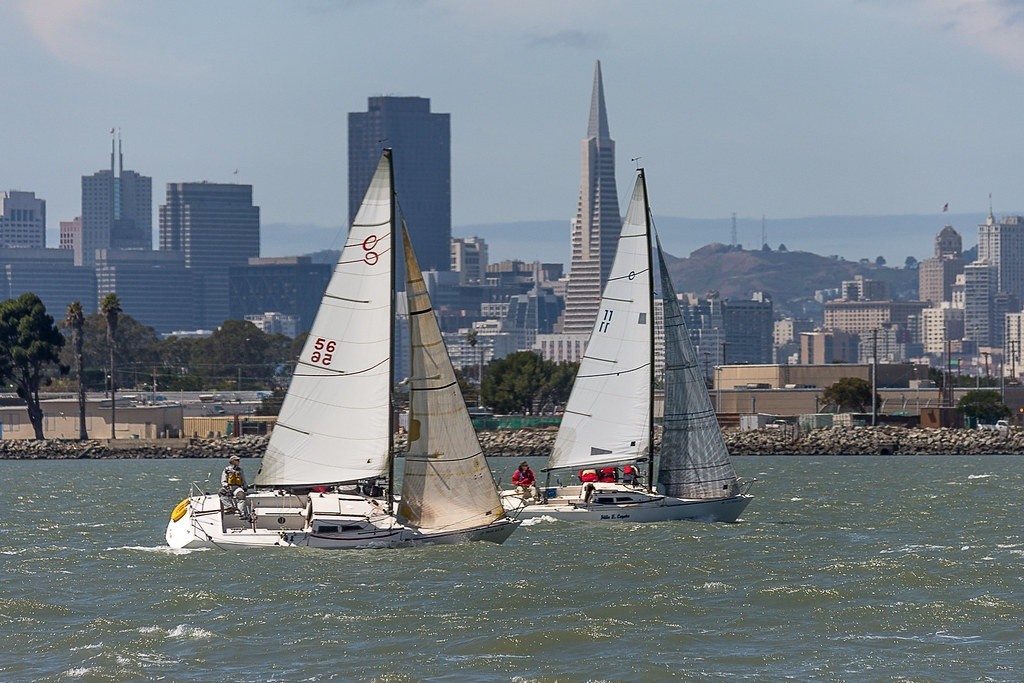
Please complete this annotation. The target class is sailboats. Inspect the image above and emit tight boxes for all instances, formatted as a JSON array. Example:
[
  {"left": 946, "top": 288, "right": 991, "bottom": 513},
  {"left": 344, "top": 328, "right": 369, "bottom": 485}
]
[
  {"left": 498, "top": 157, "right": 759, "bottom": 523},
  {"left": 166, "top": 139, "right": 530, "bottom": 552}
]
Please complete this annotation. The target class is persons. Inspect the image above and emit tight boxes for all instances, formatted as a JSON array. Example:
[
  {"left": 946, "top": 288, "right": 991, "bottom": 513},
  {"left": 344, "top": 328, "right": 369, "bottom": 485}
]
[
  {"left": 597, "top": 467, "right": 619, "bottom": 483},
  {"left": 218, "top": 455, "right": 246, "bottom": 519},
  {"left": 623, "top": 463, "right": 640, "bottom": 484},
  {"left": 578, "top": 468, "right": 600, "bottom": 483},
  {"left": 512, "top": 460, "right": 537, "bottom": 499}
]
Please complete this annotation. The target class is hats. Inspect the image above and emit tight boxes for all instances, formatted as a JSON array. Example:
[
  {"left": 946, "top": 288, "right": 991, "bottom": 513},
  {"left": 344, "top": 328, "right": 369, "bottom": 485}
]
[{"left": 229, "top": 455, "right": 240, "bottom": 463}]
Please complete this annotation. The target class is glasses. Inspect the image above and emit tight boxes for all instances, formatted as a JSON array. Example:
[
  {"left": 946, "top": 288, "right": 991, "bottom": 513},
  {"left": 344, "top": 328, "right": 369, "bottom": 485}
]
[
  {"left": 522, "top": 464, "right": 528, "bottom": 467},
  {"left": 234, "top": 459, "right": 240, "bottom": 461}
]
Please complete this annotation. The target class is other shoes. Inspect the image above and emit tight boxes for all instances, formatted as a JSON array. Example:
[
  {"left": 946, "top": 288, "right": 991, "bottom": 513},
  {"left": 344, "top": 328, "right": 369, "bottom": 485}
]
[{"left": 535, "top": 500, "right": 543, "bottom": 505}]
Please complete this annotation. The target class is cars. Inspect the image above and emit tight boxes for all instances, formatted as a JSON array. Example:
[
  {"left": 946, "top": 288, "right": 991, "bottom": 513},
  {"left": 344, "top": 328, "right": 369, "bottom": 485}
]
[{"left": 147, "top": 396, "right": 167, "bottom": 406}]
